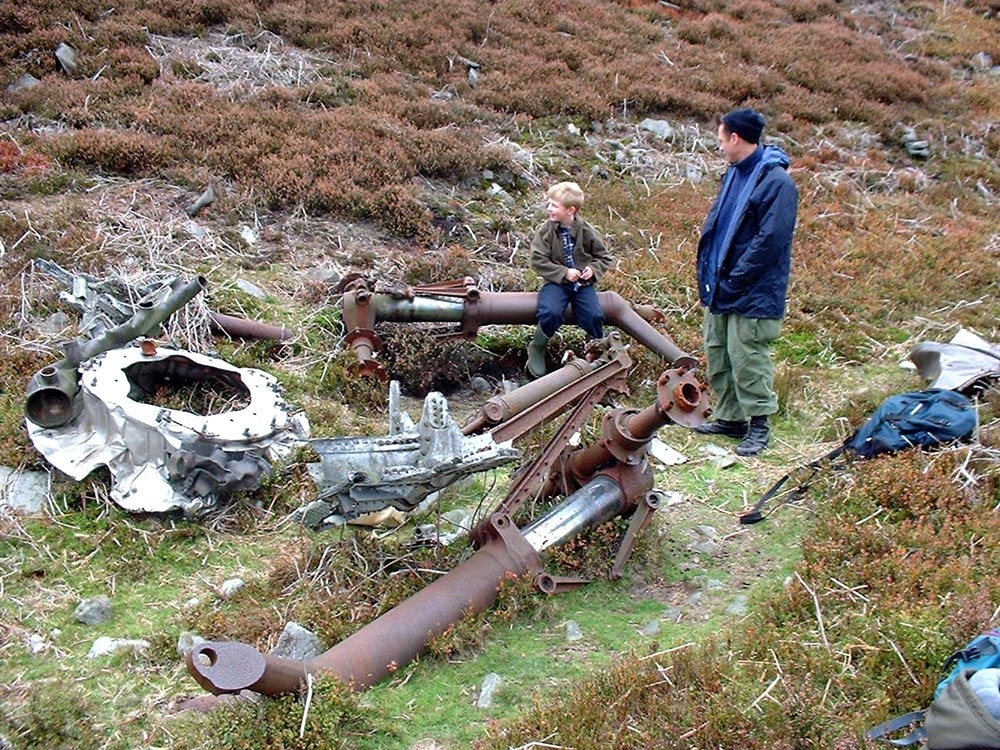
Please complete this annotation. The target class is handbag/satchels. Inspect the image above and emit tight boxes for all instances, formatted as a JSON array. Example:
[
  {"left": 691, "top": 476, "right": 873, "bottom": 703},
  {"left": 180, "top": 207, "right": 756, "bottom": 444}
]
[{"left": 847, "top": 388, "right": 976, "bottom": 459}]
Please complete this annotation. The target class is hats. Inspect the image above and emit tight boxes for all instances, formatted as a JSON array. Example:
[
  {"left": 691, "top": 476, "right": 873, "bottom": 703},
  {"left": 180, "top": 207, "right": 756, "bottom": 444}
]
[{"left": 723, "top": 110, "right": 767, "bottom": 144}]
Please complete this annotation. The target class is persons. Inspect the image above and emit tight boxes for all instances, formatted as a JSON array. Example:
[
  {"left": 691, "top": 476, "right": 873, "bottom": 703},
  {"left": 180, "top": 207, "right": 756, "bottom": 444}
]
[
  {"left": 692, "top": 108, "right": 799, "bottom": 457},
  {"left": 527, "top": 182, "right": 612, "bottom": 378}
]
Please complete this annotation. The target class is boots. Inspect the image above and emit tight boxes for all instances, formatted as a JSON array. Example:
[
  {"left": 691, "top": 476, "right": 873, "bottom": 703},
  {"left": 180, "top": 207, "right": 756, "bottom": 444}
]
[{"left": 527, "top": 321, "right": 552, "bottom": 378}]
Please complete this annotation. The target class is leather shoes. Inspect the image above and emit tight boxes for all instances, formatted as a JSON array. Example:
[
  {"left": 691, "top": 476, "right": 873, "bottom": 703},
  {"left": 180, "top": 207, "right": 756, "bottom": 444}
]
[
  {"left": 734, "top": 421, "right": 769, "bottom": 455},
  {"left": 692, "top": 417, "right": 748, "bottom": 438}
]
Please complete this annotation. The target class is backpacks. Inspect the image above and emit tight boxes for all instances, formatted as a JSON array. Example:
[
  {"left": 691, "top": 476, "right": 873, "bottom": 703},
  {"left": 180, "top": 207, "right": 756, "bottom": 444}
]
[{"left": 922, "top": 625, "right": 1000, "bottom": 750}]
